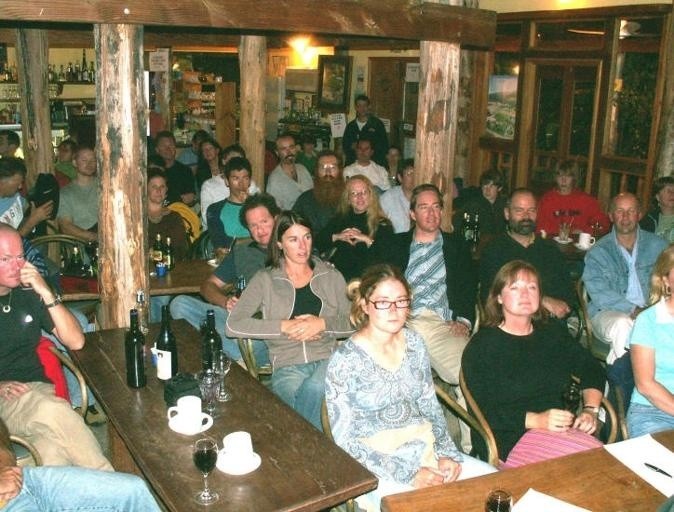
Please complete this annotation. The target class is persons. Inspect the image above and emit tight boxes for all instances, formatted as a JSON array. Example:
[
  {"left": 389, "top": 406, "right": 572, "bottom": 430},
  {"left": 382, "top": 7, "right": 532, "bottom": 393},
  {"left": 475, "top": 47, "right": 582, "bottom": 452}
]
[
  {"left": 0, "top": 129, "right": 20, "bottom": 161},
  {"left": 0, "top": 416, "right": 163, "bottom": 510},
  {"left": 0, "top": 156, "right": 54, "bottom": 281},
  {"left": 341, "top": 94, "right": 389, "bottom": 169},
  {"left": 0, "top": 222, "right": 115, "bottom": 474}
]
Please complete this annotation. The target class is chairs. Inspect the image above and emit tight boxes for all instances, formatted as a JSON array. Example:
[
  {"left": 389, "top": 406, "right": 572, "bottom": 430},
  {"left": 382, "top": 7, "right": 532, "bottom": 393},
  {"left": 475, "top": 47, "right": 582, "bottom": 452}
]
[{"left": 0, "top": 220, "right": 674, "bottom": 512}]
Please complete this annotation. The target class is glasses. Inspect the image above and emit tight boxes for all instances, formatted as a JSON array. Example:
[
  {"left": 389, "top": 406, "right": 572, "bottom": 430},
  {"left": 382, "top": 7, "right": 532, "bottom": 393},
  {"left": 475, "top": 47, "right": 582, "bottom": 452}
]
[{"left": 365, "top": 296, "right": 414, "bottom": 311}]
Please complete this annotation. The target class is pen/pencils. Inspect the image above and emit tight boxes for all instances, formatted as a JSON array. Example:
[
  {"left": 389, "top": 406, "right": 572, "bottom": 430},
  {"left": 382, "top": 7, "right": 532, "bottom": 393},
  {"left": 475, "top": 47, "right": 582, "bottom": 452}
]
[{"left": 644, "top": 463, "right": 673, "bottom": 479}]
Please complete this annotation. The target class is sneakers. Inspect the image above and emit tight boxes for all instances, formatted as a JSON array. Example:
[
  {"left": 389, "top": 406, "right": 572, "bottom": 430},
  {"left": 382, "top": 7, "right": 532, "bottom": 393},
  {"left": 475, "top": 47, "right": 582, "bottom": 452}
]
[{"left": 74, "top": 406, "right": 107, "bottom": 427}]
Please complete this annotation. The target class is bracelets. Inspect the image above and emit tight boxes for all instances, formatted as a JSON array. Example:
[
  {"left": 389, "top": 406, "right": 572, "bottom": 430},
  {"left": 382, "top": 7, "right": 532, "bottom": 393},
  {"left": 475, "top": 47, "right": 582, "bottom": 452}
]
[{"left": 42, "top": 291, "right": 62, "bottom": 310}]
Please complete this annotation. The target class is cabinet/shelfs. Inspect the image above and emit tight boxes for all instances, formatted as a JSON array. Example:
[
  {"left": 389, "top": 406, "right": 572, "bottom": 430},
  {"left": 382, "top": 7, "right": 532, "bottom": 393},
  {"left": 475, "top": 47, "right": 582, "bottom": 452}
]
[{"left": 178, "top": 77, "right": 237, "bottom": 150}]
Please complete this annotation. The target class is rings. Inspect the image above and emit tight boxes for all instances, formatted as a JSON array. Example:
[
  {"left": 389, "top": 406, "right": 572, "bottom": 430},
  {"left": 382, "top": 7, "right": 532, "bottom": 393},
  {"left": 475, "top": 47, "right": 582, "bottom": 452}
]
[{"left": 5, "top": 387, "right": 10, "bottom": 392}]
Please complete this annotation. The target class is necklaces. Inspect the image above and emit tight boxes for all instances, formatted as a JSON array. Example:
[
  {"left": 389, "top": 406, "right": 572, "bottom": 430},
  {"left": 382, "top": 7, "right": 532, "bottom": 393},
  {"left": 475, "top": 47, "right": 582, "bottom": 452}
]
[{"left": 0, "top": 288, "right": 11, "bottom": 313}]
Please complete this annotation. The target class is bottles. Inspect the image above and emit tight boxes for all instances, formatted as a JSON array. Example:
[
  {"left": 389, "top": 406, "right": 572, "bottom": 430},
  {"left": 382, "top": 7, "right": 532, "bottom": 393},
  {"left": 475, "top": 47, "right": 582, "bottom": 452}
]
[
  {"left": 236, "top": 275, "right": 246, "bottom": 298},
  {"left": 57, "top": 231, "right": 175, "bottom": 278},
  {"left": 155, "top": 305, "right": 178, "bottom": 379},
  {"left": 125, "top": 311, "right": 145, "bottom": 388},
  {"left": 134, "top": 292, "right": 149, "bottom": 334},
  {"left": 559, "top": 382, "right": 581, "bottom": 427},
  {"left": 460, "top": 211, "right": 480, "bottom": 244}
]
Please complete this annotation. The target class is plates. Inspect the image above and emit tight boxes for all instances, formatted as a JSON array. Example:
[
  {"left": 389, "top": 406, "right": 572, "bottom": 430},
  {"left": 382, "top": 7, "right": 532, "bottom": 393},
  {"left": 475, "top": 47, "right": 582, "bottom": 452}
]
[
  {"left": 168, "top": 412, "right": 211, "bottom": 435},
  {"left": 575, "top": 242, "right": 589, "bottom": 251},
  {"left": 206, "top": 258, "right": 219, "bottom": 269},
  {"left": 216, "top": 451, "right": 263, "bottom": 474},
  {"left": 554, "top": 236, "right": 574, "bottom": 244}
]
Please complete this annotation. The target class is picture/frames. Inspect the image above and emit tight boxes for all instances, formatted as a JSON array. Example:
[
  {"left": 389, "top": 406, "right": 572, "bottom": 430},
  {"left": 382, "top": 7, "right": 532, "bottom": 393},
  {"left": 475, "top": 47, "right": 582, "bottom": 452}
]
[{"left": 316, "top": 53, "right": 354, "bottom": 116}]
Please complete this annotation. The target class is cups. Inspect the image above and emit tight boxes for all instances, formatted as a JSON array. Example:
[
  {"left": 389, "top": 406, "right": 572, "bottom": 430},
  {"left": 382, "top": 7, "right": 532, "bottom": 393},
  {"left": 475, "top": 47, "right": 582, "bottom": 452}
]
[
  {"left": 164, "top": 396, "right": 204, "bottom": 428},
  {"left": 539, "top": 231, "right": 547, "bottom": 240},
  {"left": 579, "top": 232, "right": 597, "bottom": 248},
  {"left": 484, "top": 487, "right": 513, "bottom": 511},
  {"left": 560, "top": 222, "right": 570, "bottom": 240},
  {"left": 223, "top": 431, "right": 254, "bottom": 465}
]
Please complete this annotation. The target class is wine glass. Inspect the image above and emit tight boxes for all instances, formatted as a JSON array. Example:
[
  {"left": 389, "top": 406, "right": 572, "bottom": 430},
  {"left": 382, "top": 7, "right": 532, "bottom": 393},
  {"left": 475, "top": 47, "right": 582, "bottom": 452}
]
[
  {"left": 192, "top": 440, "right": 219, "bottom": 507},
  {"left": 199, "top": 310, "right": 231, "bottom": 413}
]
